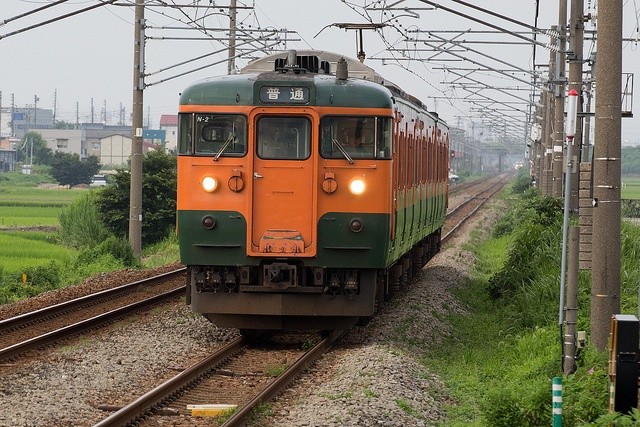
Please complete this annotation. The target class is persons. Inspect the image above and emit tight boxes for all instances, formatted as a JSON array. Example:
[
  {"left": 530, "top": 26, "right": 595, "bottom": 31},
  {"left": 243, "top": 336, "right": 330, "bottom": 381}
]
[{"left": 346, "top": 127, "right": 363, "bottom": 148}]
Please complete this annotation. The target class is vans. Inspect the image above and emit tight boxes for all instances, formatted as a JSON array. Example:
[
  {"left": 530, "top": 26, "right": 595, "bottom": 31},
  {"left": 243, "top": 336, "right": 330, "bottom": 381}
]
[{"left": 89, "top": 175, "right": 108, "bottom": 188}]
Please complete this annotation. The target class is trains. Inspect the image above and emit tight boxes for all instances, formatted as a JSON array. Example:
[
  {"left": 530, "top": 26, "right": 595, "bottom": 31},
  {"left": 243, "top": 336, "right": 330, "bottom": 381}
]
[{"left": 178, "top": 49, "right": 449, "bottom": 334}]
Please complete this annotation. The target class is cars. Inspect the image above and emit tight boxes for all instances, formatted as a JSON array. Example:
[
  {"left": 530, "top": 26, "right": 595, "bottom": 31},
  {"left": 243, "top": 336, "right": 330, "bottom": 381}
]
[{"left": 449, "top": 173, "right": 459, "bottom": 182}]
